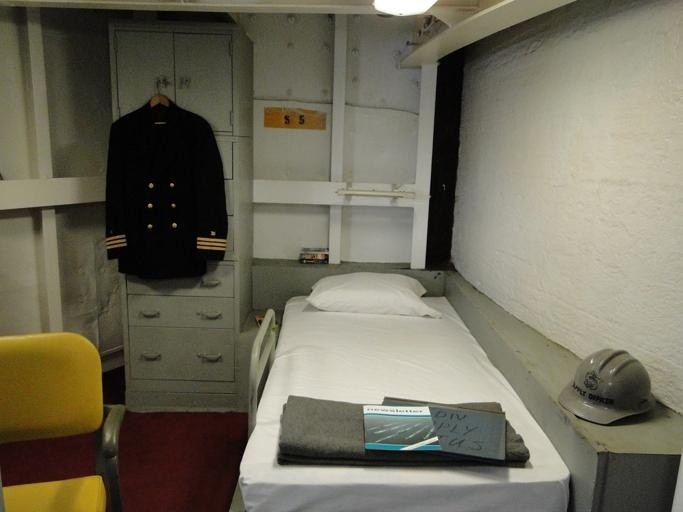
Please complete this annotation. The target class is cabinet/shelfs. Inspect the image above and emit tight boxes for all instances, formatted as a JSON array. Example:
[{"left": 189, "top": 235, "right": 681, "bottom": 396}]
[
  {"left": 108, "top": 21, "right": 253, "bottom": 413},
  {"left": 0, "top": 332, "right": 125, "bottom": 512}
]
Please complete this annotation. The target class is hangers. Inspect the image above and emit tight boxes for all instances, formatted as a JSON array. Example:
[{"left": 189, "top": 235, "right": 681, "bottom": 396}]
[{"left": 148, "top": 77, "right": 169, "bottom": 110}]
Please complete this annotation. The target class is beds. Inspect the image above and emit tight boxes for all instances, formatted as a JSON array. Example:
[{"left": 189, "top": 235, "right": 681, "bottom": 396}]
[{"left": 237, "top": 295, "right": 571, "bottom": 511}]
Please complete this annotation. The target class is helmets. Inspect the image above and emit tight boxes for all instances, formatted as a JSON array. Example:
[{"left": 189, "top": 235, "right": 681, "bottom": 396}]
[{"left": 557, "top": 348, "right": 658, "bottom": 427}]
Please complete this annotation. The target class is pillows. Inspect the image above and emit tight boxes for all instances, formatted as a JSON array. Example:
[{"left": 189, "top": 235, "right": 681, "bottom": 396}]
[{"left": 305, "top": 270, "right": 439, "bottom": 318}]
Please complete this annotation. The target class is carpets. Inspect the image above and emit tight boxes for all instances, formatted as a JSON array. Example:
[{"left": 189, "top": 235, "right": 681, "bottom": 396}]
[{"left": 0, "top": 412, "right": 248, "bottom": 512}]
